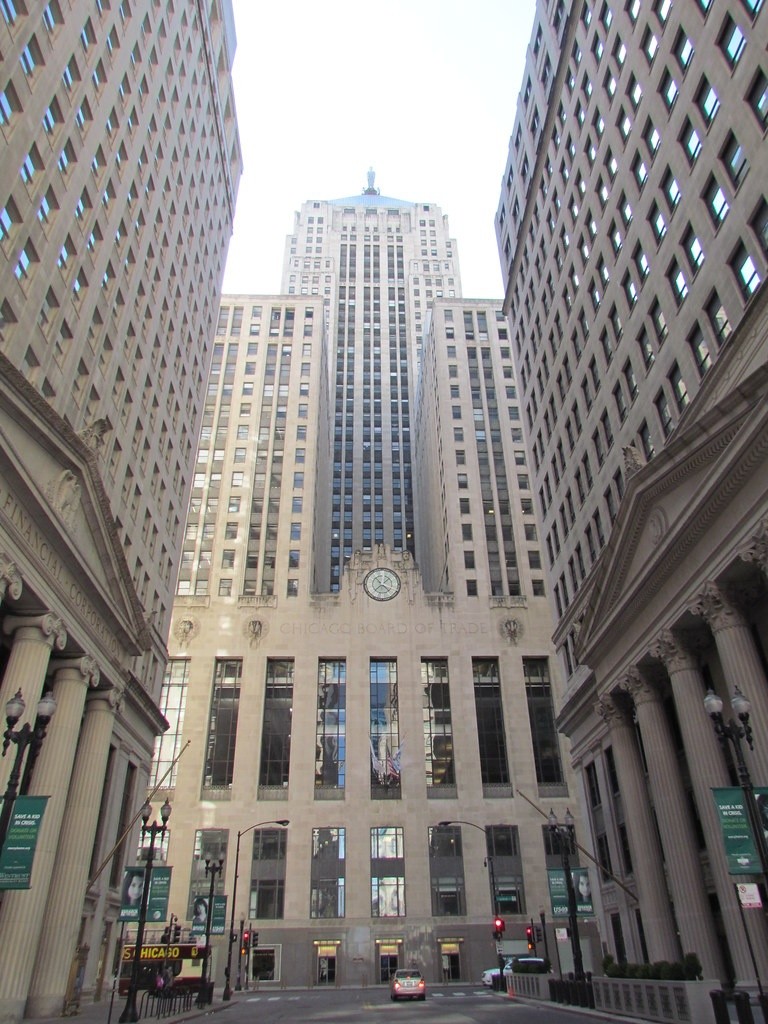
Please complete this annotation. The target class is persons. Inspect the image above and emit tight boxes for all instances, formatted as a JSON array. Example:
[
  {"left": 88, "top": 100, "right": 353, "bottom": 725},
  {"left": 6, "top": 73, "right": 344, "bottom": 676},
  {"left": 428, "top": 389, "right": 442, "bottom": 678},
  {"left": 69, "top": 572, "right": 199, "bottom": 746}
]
[
  {"left": 193, "top": 898, "right": 207, "bottom": 926},
  {"left": 573, "top": 872, "right": 592, "bottom": 904},
  {"left": 122, "top": 870, "right": 144, "bottom": 905},
  {"left": 411, "top": 972, "right": 417, "bottom": 976},
  {"left": 156, "top": 964, "right": 173, "bottom": 997}
]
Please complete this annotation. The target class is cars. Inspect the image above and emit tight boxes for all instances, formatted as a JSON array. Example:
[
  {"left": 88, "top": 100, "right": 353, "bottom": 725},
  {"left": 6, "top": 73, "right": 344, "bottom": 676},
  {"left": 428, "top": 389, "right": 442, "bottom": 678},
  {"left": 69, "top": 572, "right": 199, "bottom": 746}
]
[{"left": 388, "top": 968, "right": 426, "bottom": 1001}]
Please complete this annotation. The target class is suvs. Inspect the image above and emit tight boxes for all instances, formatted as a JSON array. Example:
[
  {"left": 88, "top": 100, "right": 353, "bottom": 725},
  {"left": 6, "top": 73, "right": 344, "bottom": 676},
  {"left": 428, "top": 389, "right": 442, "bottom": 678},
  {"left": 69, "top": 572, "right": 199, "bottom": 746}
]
[{"left": 480, "top": 957, "right": 555, "bottom": 989}]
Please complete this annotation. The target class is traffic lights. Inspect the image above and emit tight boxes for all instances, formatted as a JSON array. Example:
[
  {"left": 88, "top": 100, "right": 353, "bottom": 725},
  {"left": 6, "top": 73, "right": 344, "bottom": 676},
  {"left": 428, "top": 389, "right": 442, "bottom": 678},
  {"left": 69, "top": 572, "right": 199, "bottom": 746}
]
[
  {"left": 243, "top": 931, "right": 249, "bottom": 947},
  {"left": 528, "top": 942, "right": 535, "bottom": 950},
  {"left": 253, "top": 932, "right": 259, "bottom": 946},
  {"left": 495, "top": 918, "right": 502, "bottom": 933},
  {"left": 526, "top": 927, "right": 533, "bottom": 942},
  {"left": 533, "top": 925, "right": 543, "bottom": 943}
]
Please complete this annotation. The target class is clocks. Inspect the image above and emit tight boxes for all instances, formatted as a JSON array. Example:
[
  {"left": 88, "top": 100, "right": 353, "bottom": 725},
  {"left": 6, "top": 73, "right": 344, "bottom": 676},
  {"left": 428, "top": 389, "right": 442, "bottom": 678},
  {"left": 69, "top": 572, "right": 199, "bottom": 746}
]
[{"left": 362, "top": 567, "right": 402, "bottom": 601}]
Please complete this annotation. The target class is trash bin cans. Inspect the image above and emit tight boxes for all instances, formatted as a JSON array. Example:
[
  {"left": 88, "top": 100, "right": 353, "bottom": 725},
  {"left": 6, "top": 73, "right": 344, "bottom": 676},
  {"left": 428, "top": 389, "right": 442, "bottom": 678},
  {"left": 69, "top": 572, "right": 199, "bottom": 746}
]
[
  {"left": 492, "top": 974, "right": 505, "bottom": 991},
  {"left": 195, "top": 982, "right": 214, "bottom": 1005}
]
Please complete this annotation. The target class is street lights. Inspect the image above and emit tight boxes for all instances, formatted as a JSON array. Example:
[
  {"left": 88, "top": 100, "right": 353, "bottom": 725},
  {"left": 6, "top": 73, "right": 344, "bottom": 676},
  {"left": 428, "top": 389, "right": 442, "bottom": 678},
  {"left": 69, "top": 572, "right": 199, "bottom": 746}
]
[
  {"left": 702, "top": 684, "right": 768, "bottom": 897},
  {"left": 117, "top": 797, "right": 173, "bottom": 1024},
  {"left": 222, "top": 819, "right": 290, "bottom": 1001},
  {"left": 438, "top": 820, "right": 505, "bottom": 990},
  {"left": 173, "top": 924, "right": 181, "bottom": 943},
  {"left": 194, "top": 850, "right": 226, "bottom": 1004},
  {"left": 546, "top": 806, "right": 588, "bottom": 1008},
  {"left": 0, "top": 686, "right": 59, "bottom": 860}
]
